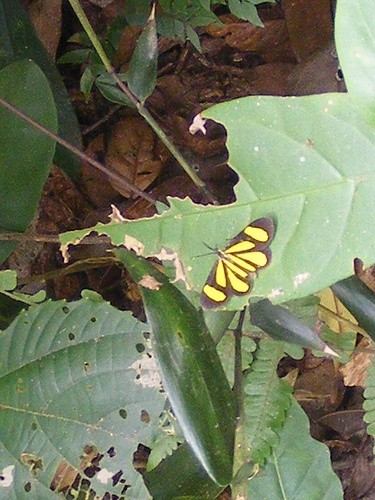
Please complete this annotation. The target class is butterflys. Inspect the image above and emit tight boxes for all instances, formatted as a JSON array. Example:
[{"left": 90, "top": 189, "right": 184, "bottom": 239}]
[{"left": 191, "top": 217, "right": 275, "bottom": 309}]
